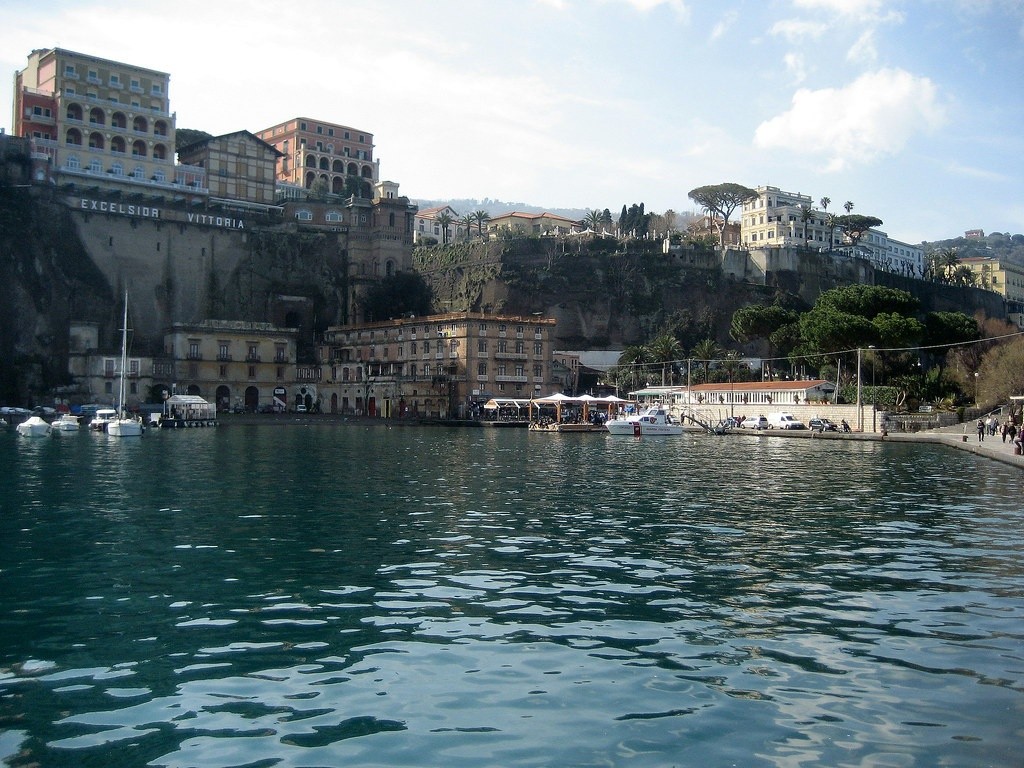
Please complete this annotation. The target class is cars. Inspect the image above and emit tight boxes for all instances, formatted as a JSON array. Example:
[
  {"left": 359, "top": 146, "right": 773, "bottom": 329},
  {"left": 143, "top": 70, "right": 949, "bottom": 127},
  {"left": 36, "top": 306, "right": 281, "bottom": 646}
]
[
  {"left": 725, "top": 417, "right": 740, "bottom": 427},
  {"left": 262, "top": 405, "right": 274, "bottom": 414},
  {"left": 297, "top": 405, "right": 307, "bottom": 413},
  {"left": 809, "top": 419, "right": 838, "bottom": 432}
]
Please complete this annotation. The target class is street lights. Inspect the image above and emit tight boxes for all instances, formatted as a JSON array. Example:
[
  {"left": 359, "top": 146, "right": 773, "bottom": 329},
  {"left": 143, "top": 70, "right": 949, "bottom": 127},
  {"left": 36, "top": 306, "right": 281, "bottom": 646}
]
[
  {"left": 973, "top": 372, "right": 979, "bottom": 396},
  {"left": 868, "top": 345, "right": 877, "bottom": 432}
]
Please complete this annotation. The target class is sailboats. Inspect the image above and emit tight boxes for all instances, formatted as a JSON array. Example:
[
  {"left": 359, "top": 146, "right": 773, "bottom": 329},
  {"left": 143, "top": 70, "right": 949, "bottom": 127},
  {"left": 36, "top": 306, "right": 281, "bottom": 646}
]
[{"left": 108, "top": 293, "right": 146, "bottom": 436}]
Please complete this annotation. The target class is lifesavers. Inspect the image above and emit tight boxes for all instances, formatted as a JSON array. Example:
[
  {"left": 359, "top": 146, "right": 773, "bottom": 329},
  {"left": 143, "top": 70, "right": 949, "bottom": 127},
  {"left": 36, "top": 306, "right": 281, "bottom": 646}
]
[{"left": 649, "top": 417, "right": 655, "bottom": 423}]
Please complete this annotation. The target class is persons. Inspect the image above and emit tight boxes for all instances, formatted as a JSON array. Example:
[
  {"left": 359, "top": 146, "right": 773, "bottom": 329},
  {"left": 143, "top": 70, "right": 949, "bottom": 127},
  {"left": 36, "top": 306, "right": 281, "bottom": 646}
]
[
  {"left": 739, "top": 416, "right": 745, "bottom": 427},
  {"left": 1008, "top": 422, "right": 1017, "bottom": 444},
  {"left": 841, "top": 420, "right": 849, "bottom": 432},
  {"left": 985, "top": 416, "right": 999, "bottom": 436},
  {"left": 577, "top": 410, "right": 608, "bottom": 428},
  {"left": 681, "top": 412, "right": 685, "bottom": 426},
  {"left": 529, "top": 415, "right": 553, "bottom": 429},
  {"left": 880, "top": 424, "right": 888, "bottom": 441},
  {"left": 977, "top": 420, "right": 985, "bottom": 441},
  {"left": 1015, "top": 424, "right": 1024, "bottom": 455},
  {"left": 1000, "top": 421, "right": 1008, "bottom": 443}
]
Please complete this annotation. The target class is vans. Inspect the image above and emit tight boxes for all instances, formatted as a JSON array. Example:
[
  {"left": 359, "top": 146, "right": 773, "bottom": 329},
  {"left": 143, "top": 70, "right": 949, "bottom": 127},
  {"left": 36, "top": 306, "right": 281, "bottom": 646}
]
[
  {"left": 741, "top": 415, "right": 768, "bottom": 429},
  {"left": 767, "top": 411, "right": 805, "bottom": 430}
]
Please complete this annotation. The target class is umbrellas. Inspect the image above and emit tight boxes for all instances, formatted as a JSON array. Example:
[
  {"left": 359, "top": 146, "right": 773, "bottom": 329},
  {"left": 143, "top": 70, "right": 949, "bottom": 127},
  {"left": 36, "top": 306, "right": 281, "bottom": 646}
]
[{"left": 531, "top": 392, "right": 628, "bottom": 404}]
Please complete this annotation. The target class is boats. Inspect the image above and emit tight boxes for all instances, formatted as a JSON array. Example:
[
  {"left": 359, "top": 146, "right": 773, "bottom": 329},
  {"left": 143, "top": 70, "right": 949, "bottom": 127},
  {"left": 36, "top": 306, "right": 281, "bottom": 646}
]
[
  {"left": 59, "top": 415, "right": 80, "bottom": 431},
  {"left": 604, "top": 409, "right": 685, "bottom": 434},
  {"left": 16, "top": 415, "right": 53, "bottom": 437},
  {"left": 50, "top": 421, "right": 63, "bottom": 429},
  {"left": 89, "top": 409, "right": 117, "bottom": 432}
]
